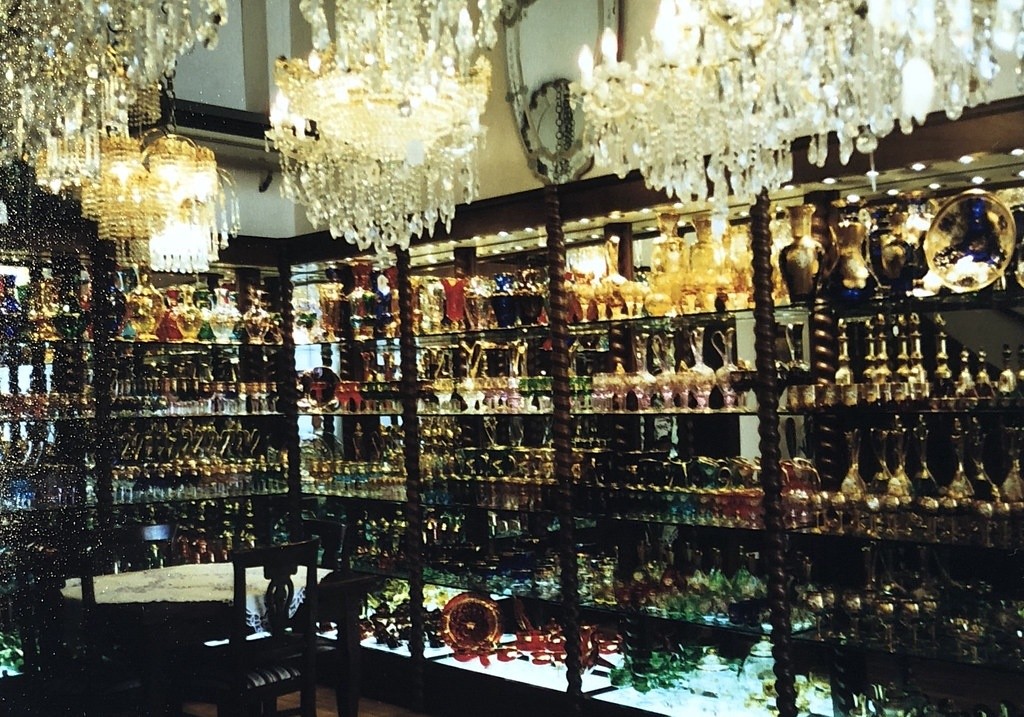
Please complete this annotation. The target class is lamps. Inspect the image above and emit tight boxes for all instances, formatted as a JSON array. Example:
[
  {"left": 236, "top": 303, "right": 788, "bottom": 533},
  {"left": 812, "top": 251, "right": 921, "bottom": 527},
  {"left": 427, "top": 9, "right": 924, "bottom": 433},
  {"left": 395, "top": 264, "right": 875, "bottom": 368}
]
[
  {"left": 567, "top": 0, "right": 1024, "bottom": 216},
  {"left": 2, "top": 0, "right": 239, "bottom": 275},
  {"left": 263, "top": 0, "right": 506, "bottom": 258}
]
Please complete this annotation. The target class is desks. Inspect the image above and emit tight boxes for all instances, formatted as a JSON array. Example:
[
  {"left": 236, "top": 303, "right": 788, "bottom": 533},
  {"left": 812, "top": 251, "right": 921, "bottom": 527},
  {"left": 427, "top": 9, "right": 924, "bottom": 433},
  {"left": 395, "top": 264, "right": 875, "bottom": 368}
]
[{"left": 59, "top": 561, "right": 334, "bottom": 716}]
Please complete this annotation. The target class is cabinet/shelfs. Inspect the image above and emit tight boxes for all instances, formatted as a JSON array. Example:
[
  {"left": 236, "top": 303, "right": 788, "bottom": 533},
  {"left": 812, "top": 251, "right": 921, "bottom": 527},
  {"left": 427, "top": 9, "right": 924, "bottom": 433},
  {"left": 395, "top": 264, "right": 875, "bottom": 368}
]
[{"left": 0, "top": 95, "right": 1024, "bottom": 717}]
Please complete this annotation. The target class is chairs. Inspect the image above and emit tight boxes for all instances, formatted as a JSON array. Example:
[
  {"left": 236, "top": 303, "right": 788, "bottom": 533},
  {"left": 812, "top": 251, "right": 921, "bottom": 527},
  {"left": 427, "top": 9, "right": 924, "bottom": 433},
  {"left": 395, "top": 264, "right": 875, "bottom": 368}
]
[
  {"left": 171, "top": 542, "right": 320, "bottom": 717},
  {"left": 124, "top": 521, "right": 202, "bottom": 572},
  {"left": 15, "top": 548, "right": 156, "bottom": 716},
  {"left": 248, "top": 517, "right": 351, "bottom": 716}
]
